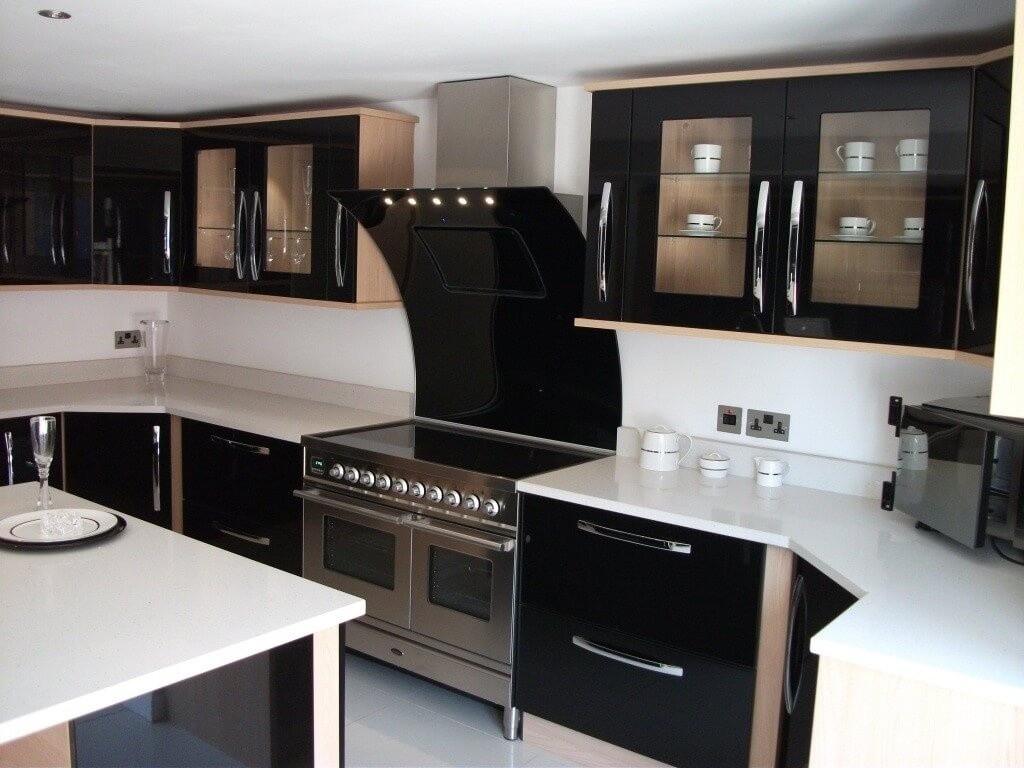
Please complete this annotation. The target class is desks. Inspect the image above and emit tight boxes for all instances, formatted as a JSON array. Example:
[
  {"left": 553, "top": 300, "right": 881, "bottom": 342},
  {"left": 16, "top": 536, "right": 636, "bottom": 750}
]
[{"left": 0, "top": 480, "right": 367, "bottom": 768}]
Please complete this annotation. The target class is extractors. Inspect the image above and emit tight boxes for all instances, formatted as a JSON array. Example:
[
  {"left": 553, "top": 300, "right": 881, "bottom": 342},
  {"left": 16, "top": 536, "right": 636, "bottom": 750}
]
[{"left": 327, "top": 75, "right": 623, "bottom": 447}]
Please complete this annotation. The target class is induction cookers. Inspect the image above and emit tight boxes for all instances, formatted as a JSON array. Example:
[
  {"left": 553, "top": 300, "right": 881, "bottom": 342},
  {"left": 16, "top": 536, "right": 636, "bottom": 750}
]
[{"left": 302, "top": 420, "right": 608, "bottom": 530}]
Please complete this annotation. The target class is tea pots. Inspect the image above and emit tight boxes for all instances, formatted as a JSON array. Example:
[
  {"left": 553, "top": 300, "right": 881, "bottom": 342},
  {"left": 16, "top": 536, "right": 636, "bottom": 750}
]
[{"left": 634, "top": 424, "right": 692, "bottom": 472}]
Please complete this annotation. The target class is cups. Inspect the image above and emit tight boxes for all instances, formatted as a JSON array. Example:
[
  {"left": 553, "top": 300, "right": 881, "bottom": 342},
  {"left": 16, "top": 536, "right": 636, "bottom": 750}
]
[
  {"left": 139, "top": 319, "right": 170, "bottom": 381},
  {"left": 690, "top": 143, "right": 721, "bottom": 173},
  {"left": 903, "top": 218, "right": 924, "bottom": 236},
  {"left": 699, "top": 452, "right": 730, "bottom": 479},
  {"left": 753, "top": 457, "right": 791, "bottom": 487},
  {"left": 686, "top": 214, "right": 722, "bottom": 231},
  {"left": 835, "top": 141, "right": 875, "bottom": 171},
  {"left": 839, "top": 217, "right": 876, "bottom": 235},
  {"left": 895, "top": 139, "right": 927, "bottom": 171}
]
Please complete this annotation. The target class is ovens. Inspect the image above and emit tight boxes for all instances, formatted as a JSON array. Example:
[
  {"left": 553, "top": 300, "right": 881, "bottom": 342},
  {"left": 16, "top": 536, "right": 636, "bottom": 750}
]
[{"left": 293, "top": 485, "right": 518, "bottom": 740}]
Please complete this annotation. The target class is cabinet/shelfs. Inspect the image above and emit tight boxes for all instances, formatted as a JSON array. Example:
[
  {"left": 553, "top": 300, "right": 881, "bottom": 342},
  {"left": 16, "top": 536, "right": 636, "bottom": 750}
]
[
  {"left": 0, "top": 107, "right": 93, "bottom": 293},
  {"left": 955, "top": 41, "right": 1015, "bottom": 370},
  {"left": 183, "top": 105, "right": 421, "bottom": 314},
  {"left": 0, "top": 411, "right": 63, "bottom": 491},
  {"left": 519, "top": 484, "right": 1024, "bottom": 768},
  {"left": 175, "top": 418, "right": 303, "bottom": 576},
  {"left": 572, "top": 54, "right": 976, "bottom": 362},
  {"left": 93, "top": 111, "right": 181, "bottom": 295},
  {"left": 60, "top": 411, "right": 175, "bottom": 530}
]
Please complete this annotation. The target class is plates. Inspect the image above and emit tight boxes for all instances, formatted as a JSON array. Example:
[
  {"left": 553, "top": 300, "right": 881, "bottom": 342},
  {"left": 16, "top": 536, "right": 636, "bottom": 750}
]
[
  {"left": 677, "top": 229, "right": 721, "bottom": 237},
  {"left": 829, "top": 234, "right": 878, "bottom": 241},
  {"left": 892, "top": 236, "right": 923, "bottom": 243},
  {"left": 0, "top": 510, "right": 127, "bottom": 549}
]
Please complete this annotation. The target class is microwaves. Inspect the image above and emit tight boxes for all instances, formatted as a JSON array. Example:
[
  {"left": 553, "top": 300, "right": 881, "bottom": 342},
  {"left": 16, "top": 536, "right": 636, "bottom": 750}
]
[{"left": 891, "top": 396, "right": 1024, "bottom": 554}]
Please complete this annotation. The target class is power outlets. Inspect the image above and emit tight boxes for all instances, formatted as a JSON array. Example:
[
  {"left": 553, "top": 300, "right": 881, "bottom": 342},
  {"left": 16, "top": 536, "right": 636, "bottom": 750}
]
[
  {"left": 114, "top": 330, "right": 142, "bottom": 349},
  {"left": 745, "top": 408, "right": 790, "bottom": 442}
]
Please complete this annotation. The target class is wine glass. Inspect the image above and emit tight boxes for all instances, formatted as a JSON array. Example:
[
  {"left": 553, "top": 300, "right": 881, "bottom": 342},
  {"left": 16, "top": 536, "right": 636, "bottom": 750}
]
[
  {"left": 228, "top": 167, "right": 236, "bottom": 230},
  {"left": 301, "top": 164, "right": 312, "bottom": 231},
  {"left": 30, "top": 415, "right": 56, "bottom": 511},
  {"left": 290, "top": 239, "right": 307, "bottom": 274},
  {"left": 220, "top": 233, "right": 235, "bottom": 267}
]
